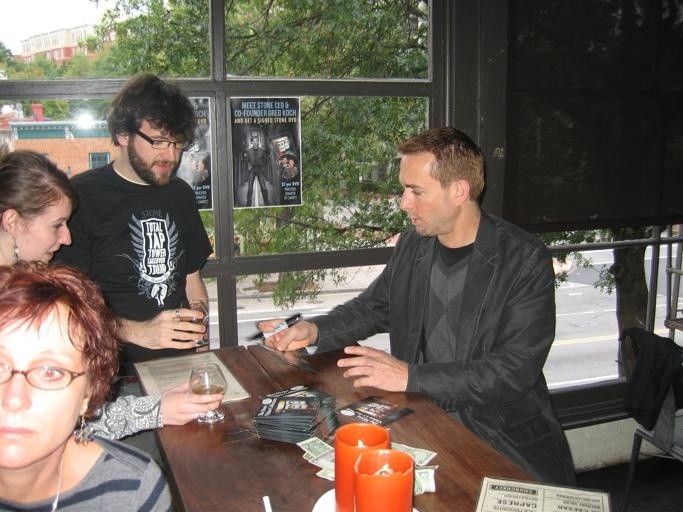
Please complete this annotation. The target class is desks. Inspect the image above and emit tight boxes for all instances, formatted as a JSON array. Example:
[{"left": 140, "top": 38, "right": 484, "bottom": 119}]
[
  {"left": 127, "top": 337, "right": 544, "bottom": 512},
  {"left": 662, "top": 315, "right": 682, "bottom": 334}
]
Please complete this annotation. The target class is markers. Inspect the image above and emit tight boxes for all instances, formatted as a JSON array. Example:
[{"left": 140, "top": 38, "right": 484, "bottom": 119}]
[{"left": 245, "top": 314, "right": 302, "bottom": 341}]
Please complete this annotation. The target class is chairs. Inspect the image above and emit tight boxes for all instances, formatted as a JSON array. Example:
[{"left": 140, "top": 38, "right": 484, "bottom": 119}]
[{"left": 616, "top": 326, "right": 681, "bottom": 510}]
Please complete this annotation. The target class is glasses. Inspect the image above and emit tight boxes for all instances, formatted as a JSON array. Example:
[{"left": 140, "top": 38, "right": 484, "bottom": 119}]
[
  {"left": 2, "top": 363, "right": 89, "bottom": 389},
  {"left": 133, "top": 128, "right": 190, "bottom": 150}
]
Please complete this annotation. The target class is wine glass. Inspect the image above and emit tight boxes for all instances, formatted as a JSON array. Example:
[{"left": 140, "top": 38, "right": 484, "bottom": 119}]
[
  {"left": 186, "top": 364, "right": 226, "bottom": 425},
  {"left": 179, "top": 298, "right": 211, "bottom": 350}
]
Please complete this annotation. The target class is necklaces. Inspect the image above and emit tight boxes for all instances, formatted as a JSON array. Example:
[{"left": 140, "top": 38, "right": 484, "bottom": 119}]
[{"left": 52, "top": 456, "right": 63, "bottom": 512}]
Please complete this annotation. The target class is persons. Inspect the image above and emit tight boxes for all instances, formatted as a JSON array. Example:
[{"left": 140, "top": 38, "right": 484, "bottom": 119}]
[
  {"left": 246, "top": 136, "right": 271, "bottom": 206},
  {"left": 1, "top": 260, "right": 172, "bottom": 512},
  {"left": 256, "top": 126, "right": 577, "bottom": 487},
  {"left": 0, "top": 150, "right": 223, "bottom": 441},
  {"left": 48, "top": 74, "right": 213, "bottom": 477}
]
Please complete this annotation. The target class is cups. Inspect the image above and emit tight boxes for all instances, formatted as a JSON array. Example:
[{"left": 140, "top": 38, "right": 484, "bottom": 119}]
[{"left": 333, "top": 422, "right": 415, "bottom": 510}]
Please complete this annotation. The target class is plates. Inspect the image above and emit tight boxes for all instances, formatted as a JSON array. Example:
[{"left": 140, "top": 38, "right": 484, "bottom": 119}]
[{"left": 311, "top": 487, "right": 421, "bottom": 512}]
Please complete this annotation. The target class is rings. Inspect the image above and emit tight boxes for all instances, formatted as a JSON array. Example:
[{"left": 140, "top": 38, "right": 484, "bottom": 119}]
[{"left": 176, "top": 309, "right": 179, "bottom": 318}]
